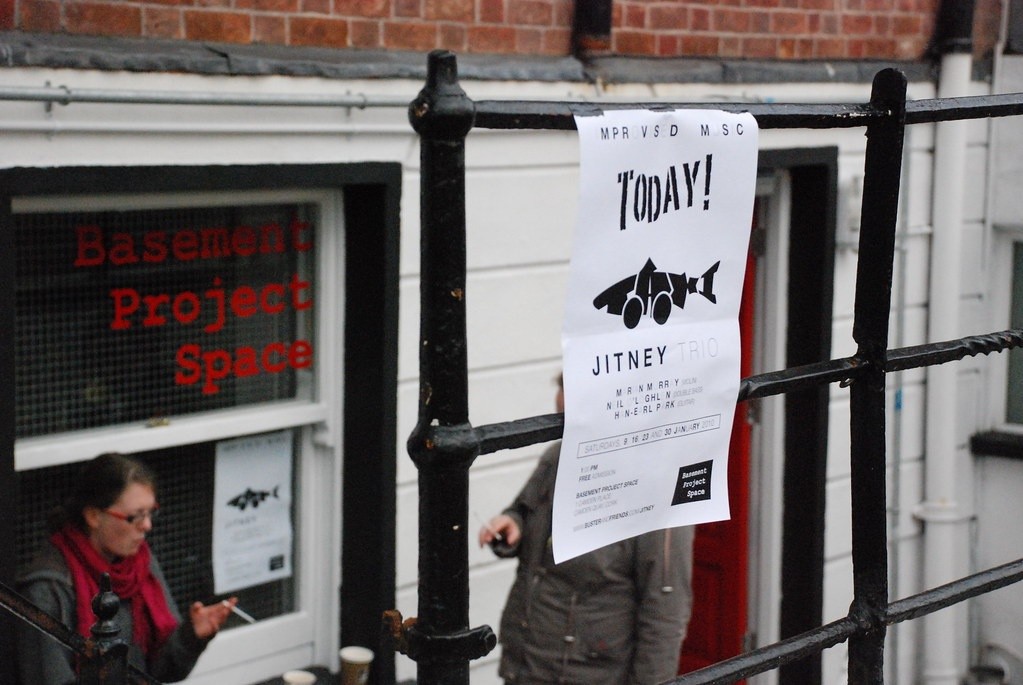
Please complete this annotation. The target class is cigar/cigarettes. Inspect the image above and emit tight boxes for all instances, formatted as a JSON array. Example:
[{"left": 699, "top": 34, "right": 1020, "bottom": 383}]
[
  {"left": 220, "top": 599, "right": 256, "bottom": 624},
  {"left": 480, "top": 520, "right": 502, "bottom": 540}
]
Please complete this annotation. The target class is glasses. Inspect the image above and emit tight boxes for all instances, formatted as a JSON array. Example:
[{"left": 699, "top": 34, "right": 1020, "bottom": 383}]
[{"left": 98, "top": 502, "right": 161, "bottom": 524}]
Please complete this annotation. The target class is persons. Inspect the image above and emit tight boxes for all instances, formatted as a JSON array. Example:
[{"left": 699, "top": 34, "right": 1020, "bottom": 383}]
[
  {"left": 475, "top": 367, "right": 694, "bottom": 684},
  {"left": 13, "top": 452, "right": 238, "bottom": 683}
]
